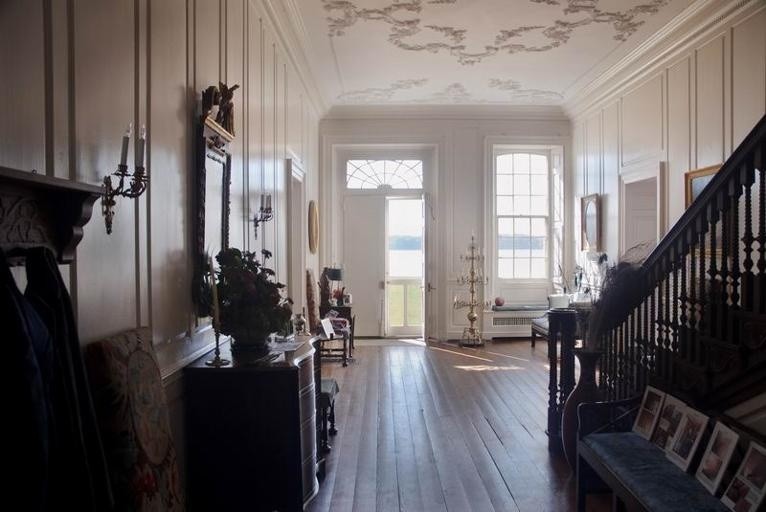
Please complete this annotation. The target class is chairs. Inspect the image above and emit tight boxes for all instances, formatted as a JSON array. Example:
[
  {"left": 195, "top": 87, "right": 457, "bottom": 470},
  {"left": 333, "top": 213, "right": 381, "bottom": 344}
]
[
  {"left": 574, "top": 374, "right": 766, "bottom": 512},
  {"left": 530, "top": 293, "right": 598, "bottom": 359},
  {"left": 305, "top": 268, "right": 350, "bottom": 368}
]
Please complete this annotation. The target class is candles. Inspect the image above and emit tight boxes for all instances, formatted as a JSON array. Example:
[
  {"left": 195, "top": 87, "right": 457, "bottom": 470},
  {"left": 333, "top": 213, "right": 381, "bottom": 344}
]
[
  {"left": 260, "top": 194, "right": 271, "bottom": 209},
  {"left": 119, "top": 135, "right": 144, "bottom": 167}
]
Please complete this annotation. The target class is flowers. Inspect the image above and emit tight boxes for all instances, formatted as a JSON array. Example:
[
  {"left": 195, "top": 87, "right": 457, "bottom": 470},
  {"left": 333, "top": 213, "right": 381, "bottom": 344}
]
[{"left": 196, "top": 248, "right": 293, "bottom": 341}]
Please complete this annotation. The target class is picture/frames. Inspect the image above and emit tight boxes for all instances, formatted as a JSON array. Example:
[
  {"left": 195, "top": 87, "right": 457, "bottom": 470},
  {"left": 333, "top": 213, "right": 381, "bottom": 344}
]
[
  {"left": 190, "top": 135, "right": 234, "bottom": 318},
  {"left": 579, "top": 193, "right": 602, "bottom": 253},
  {"left": 683, "top": 162, "right": 727, "bottom": 257}
]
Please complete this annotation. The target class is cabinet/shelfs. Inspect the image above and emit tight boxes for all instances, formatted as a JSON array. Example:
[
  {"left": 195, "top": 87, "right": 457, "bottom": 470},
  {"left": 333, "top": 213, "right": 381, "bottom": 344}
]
[
  {"left": 181, "top": 334, "right": 325, "bottom": 512},
  {"left": 0, "top": 163, "right": 106, "bottom": 267}
]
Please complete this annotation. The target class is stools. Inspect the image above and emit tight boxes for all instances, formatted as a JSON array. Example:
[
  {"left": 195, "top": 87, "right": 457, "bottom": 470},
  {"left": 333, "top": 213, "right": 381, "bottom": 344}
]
[{"left": 321, "top": 378, "right": 339, "bottom": 454}]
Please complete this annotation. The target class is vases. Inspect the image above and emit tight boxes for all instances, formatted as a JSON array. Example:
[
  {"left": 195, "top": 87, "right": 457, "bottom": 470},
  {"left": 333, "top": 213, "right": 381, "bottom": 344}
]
[{"left": 229, "top": 342, "right": 272, "bottom": 367}]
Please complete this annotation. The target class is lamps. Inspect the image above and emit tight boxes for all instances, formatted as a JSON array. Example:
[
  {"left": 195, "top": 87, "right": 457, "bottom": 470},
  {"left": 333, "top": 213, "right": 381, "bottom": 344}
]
[{"left": 327, "top": 269, "right": 344, "bottom": 306}]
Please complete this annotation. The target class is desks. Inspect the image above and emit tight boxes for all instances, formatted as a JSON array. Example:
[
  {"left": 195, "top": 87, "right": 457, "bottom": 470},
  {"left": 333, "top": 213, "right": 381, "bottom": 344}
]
[{"left": 318, "top": 302, "right": 355, "bottom": 357}]
